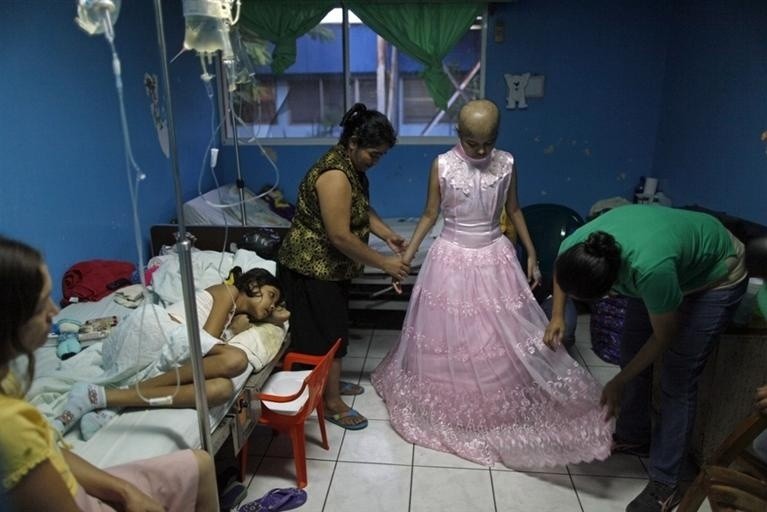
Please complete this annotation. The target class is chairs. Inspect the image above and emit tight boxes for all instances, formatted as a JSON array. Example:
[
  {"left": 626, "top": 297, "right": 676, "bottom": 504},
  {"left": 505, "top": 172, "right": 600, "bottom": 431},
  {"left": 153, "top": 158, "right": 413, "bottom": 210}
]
[{"left": 239, "top": 333, "right": 343, "bottom": 490}]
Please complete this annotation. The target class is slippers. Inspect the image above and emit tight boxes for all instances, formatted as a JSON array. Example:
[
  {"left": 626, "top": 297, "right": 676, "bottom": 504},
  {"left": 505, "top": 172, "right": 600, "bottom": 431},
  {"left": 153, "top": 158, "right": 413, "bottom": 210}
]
[
  {"left": 239, "top": 487, "right": 307, "bottom": 512},
  {"left": 324, "top": 407, "right": 368, "bottom": 430},
  {"left": 339, "top": 382, "right": 364, "bottom": 395}
]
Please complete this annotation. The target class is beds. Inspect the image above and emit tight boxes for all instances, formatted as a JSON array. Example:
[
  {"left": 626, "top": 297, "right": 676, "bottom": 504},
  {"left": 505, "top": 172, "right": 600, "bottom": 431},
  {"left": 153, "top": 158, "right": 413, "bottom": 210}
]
[
  {"left": 21, "top": 266, "right": 292, "bottom": 460},
  {"left": 170, "top": 178, "right": 437, "bottom": 312}
]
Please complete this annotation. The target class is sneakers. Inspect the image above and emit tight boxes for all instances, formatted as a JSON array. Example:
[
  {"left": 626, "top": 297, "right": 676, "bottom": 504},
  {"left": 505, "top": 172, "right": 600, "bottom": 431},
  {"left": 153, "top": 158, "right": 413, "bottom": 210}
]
[
  {"left": 611, "top": 433, "right": 652, "bottom": 458},
  {"left": 626, "top": 479, "right": 682, "bottom": 512}
]
